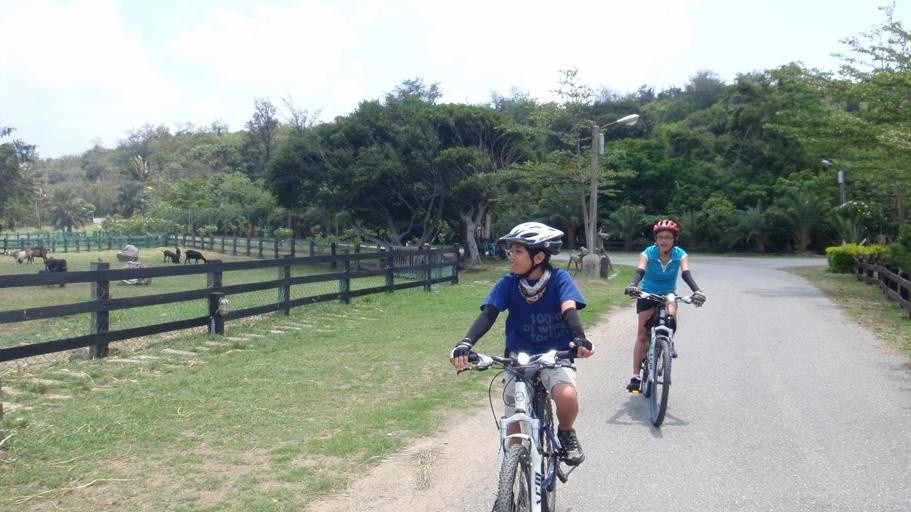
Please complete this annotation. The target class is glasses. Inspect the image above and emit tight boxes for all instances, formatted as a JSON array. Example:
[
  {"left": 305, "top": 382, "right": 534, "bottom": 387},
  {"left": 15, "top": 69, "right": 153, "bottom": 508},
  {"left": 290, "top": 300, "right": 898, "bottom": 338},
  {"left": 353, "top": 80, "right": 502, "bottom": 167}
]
[{"left": 655, "top": 235, "right": 675, "bottom": 239}]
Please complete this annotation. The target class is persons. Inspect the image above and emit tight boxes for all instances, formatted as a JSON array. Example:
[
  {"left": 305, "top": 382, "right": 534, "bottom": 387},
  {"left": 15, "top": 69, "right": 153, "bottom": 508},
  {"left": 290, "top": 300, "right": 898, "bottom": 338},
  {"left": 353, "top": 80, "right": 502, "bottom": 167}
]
[
  {"left": 451, "top": 222, "right": 604, "bottom": 466},
  {"left": 624, "top": 220, "right": 707, "bottom": 392}
]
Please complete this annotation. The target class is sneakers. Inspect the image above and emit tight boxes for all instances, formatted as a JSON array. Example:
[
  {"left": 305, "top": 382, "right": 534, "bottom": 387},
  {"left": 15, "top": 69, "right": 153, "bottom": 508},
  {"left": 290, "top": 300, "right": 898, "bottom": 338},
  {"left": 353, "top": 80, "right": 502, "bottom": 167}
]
[
  {"left": 557, "top": 427, "right": 585, "bottom": 466},
  {"left": 626, "top": 378, "right": 641, "bottom": 393}
]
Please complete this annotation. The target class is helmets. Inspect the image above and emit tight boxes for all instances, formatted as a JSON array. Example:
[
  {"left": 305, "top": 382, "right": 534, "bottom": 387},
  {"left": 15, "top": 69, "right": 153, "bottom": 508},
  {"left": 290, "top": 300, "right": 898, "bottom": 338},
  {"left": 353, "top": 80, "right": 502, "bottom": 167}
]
[
  {"left": 653, "top": 219, "right": 680, "bottom": 240},
  {"left": 499, "top": 221, "right": 565, "bottom": 255}
]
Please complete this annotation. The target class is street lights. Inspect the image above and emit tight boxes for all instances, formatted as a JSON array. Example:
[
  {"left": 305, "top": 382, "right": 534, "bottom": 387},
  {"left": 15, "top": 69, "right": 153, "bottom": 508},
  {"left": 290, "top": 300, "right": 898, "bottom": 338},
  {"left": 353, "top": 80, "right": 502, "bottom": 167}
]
[
  {"left": 821, "top": 158, "right": 847, "bottom": 205},
  {"left": 579, "top": 113, "right": 644, "bottom": 280}
]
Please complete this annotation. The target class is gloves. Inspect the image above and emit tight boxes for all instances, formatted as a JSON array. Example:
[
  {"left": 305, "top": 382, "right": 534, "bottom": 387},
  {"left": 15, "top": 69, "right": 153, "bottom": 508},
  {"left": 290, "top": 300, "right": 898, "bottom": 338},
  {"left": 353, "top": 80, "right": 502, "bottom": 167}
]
[
  {"left": 692, "top": 290, "right": 706, "bottom": 304},
  {"left": 567, "top": 336, "right": 591, "bottom": 350},
  {"left": 625, "top": 286, "right": 639, "bottom": 296},
  {"left": 451, "top": 343, "right": 471, "bottom": 358}
]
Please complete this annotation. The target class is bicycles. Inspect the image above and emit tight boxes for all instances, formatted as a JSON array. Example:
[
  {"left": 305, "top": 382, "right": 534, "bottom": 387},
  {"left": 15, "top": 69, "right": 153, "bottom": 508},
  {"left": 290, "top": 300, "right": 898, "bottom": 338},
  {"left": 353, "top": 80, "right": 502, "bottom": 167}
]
[
  {"left": 446, "top": 346, "right": 588, "bottom": 512},
  {"left": 618, "top": 281, "right": 701, "bottom": 430}
]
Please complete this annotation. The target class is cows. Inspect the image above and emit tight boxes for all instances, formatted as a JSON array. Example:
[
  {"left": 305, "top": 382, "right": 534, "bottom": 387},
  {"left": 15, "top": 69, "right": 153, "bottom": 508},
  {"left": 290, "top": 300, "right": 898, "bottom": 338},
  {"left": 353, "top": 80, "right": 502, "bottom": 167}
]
[
  {"left": 45, "top": 256, "right": 67, "bottom": 287},
  {"left": 4, "top": 247, "right": 48, "bottom": 264}
]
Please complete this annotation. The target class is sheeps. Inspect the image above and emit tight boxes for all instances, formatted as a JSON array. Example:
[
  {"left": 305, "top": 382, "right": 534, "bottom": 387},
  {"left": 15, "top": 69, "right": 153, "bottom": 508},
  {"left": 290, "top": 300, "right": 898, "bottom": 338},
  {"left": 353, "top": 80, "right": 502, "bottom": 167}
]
[
  {"left": 184, "top": 250, "right": 207, "bottom": 265},
  {"left": 161, "top": 247, "right": 181, "bottom": 264}
]
